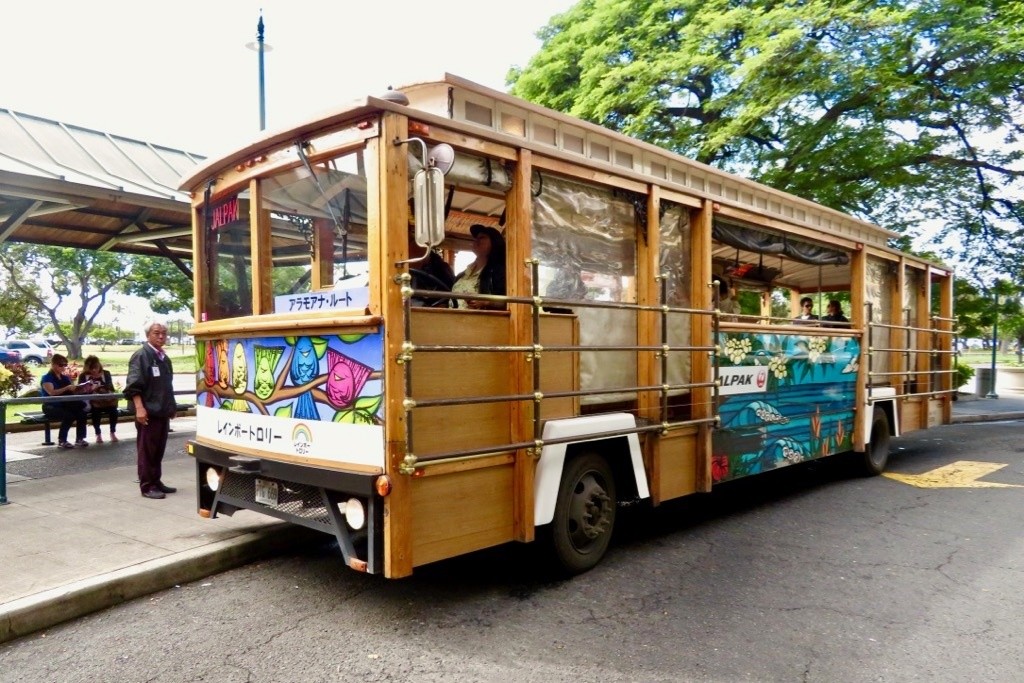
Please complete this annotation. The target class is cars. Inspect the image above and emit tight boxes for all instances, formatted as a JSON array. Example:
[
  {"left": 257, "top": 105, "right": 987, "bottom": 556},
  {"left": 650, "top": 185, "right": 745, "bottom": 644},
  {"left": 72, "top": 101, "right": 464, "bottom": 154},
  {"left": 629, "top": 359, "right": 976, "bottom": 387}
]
[{"left": 45, "top": 337, "right": 135, "bottom": 346}]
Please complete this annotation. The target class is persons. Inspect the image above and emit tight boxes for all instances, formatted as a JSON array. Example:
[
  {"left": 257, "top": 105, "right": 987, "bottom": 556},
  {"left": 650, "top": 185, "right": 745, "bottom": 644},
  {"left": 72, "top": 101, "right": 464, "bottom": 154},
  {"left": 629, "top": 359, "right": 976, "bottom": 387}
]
[
  {"left": 822, "top": 300, "right": 851, "bottom": 329},
  {"left": 41, "top": 354, "right": 94, "bottom": 448},
  {"left": 409, "top": 241, "right": 454, "bottom": 301},
  {"left": 793, "top": 297, "right": 819, "bottom": 325},
  {"left": 713, "top": 278, "right": 741, "bottom": 322},
  {"left": 449, "top": 225, "right": 507, "bottom": 311},
  {"left": 122, "top": 320, "right": 177, "bottom": 498},
  {"left": 77, "top": 355, "right": 118, "bottom": 442}
]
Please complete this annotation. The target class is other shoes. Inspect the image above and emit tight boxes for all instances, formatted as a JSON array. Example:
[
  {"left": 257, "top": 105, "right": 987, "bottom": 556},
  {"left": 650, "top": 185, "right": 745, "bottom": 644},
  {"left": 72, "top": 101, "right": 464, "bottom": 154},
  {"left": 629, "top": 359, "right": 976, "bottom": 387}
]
[
  {"left": 109, "top": 432, "right": 117, "bottom": 441},
  {"left": 144, "top": 489, "right": 166, "bottom": 498},
  {"left": 160, "top": 486, "right": 176, "bottom": 493},
  {"left": 96, "top": 434, "right": 103, "bottom": 443}
]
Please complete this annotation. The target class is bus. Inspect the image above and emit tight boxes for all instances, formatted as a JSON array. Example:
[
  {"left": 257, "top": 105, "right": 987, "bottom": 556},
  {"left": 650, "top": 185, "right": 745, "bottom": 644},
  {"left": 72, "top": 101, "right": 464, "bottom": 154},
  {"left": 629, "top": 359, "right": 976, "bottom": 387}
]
[{"left": 173, "top": 71, "right": 962, "bottom": 581}]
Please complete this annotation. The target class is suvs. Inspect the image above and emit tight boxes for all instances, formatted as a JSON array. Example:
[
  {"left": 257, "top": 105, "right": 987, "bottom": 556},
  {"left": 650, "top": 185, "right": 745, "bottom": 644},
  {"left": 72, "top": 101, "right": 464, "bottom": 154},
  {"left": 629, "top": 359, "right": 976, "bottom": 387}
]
[{"left": 0, "top": 338, "right": 55, "bottom": 368}]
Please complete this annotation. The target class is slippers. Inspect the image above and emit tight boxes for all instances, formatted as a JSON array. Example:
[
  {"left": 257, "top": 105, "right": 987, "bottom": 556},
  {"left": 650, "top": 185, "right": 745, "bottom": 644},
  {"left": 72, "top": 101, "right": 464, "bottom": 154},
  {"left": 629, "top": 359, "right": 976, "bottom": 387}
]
[
  {"left": 76, "top": 441, "right": 88, "bottom": 446},
  {"left": 59, "top": 441, "right": 73, "bottom": 448}
]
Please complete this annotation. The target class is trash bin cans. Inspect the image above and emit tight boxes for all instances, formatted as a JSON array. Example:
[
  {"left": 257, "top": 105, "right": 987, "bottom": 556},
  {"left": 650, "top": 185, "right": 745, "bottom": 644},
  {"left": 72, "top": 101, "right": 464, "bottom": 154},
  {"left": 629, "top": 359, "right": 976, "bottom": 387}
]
[{"left": 975, "top": 367, "right": 997, "bottom": 397}]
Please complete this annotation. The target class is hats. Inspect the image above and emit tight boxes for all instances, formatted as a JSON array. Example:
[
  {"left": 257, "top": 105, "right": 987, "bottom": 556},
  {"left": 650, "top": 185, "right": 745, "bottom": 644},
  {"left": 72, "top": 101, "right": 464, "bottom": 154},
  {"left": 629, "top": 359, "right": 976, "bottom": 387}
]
[{"left": 470, "top": 224, "right": 499, "bottom": 245}]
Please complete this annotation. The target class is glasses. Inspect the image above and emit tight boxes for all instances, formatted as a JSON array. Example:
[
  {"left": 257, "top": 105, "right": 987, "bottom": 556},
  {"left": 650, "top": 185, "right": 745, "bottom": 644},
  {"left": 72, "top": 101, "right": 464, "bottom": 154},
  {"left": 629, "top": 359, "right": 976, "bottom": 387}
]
[
  {"left": 804, "top": 304, "right": 813, "bottom": 308},
  {"left": 57, "top": 363, "right": 68, "bottom": 367}
]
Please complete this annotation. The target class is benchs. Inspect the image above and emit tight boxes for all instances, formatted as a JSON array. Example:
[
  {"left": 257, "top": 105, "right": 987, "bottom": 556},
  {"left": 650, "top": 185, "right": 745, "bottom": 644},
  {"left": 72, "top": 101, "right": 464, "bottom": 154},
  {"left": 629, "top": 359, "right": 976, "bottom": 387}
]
[{"left": 14, "top": 404, "right": 194, "bottom": 444}]
